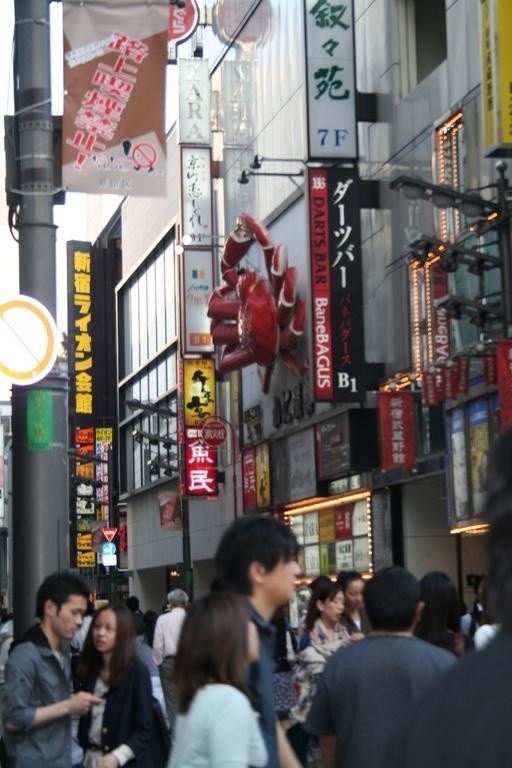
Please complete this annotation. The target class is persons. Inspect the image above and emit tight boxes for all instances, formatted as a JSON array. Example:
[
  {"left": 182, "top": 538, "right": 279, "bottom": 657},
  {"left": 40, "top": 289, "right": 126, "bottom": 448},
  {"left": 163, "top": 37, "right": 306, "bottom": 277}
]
[{"left": 1, "top": 515, "right": 512, "bottom": 768}]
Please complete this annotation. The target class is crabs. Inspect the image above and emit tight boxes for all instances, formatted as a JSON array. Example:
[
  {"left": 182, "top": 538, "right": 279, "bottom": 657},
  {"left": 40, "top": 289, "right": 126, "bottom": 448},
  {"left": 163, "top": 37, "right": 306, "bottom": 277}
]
[{"left": 206, "top": 211, "right": 310, "bottom": 394}]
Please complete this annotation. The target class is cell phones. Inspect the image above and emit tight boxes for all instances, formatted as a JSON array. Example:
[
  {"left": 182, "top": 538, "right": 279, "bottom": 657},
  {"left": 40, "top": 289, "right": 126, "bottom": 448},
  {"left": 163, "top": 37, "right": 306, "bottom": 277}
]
[{"left": 92, "top": 685, "right": 120, "bottom": 698}]
